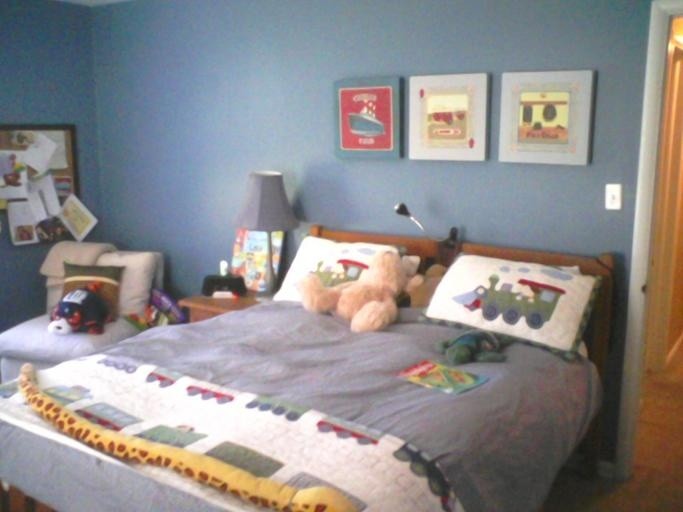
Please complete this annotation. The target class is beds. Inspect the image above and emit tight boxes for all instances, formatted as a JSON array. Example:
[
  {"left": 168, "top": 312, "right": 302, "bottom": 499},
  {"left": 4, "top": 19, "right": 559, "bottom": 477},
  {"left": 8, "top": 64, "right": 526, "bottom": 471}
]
[{"left": 1, "top": 225, "right": 614, "bottom": 512}]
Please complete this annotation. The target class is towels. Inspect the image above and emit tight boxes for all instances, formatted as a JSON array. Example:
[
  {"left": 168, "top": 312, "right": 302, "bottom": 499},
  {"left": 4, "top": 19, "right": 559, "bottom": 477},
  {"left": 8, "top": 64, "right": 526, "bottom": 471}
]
[{"left": 39, "top": 240, "right": 118, "bottom": 277}]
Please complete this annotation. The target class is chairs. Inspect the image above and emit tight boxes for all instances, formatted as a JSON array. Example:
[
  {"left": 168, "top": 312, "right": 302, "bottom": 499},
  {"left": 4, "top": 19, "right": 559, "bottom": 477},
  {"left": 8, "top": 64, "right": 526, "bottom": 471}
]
[{"left": 0, "top": 252, "right": 164, "bottom": 384}]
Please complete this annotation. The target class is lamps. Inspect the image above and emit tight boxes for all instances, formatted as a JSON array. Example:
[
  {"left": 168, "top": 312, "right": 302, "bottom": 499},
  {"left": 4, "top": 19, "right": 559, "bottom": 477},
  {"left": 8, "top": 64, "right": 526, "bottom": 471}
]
[
  {"left": 237, "top": 171, "right": 299, "bottom": 302},
  {"left": 394, "top": 202, "right": 458, "bottom": 248}
]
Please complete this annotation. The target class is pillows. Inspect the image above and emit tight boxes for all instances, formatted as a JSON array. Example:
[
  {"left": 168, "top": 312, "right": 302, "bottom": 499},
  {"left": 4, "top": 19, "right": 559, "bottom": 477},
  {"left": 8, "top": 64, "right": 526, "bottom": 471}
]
[
  {"left": 272, "top": 235, "right": 402, "bottom": 306},
  {"left": 61, "top": 261, "right": 125, "bottom": 322},
  {"left": 418, "top": 250, "right": 606, "bottom": 364}
]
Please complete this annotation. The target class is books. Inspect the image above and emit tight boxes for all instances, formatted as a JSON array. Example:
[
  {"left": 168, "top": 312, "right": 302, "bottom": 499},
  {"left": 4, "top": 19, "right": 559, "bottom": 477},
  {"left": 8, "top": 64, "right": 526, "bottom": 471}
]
[
  {"left": 383, "top": 360, "right": 490, "bottom": 395},
  {"left": 231, "top": 228, "right": 286, "bottom": 293}
]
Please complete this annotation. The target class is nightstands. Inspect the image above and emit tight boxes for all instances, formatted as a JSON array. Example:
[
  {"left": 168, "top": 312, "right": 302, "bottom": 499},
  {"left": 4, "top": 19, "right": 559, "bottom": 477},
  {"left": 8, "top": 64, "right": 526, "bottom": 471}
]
[{"left": 176, "top": 294, "right": 261, "bottom": 322}]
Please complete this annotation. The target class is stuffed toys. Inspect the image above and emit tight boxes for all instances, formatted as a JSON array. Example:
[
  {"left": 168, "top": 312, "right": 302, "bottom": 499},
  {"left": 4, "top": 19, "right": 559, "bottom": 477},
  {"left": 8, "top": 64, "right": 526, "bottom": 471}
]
[
  {"left": 301, "top": 250, "right": 425, "bottom": 334},
  {"left": 436, "top": 327, "right": 519, "bottom": 367},
  {"left": 46, "top": 279, "right": 115, "bottom": 335}
]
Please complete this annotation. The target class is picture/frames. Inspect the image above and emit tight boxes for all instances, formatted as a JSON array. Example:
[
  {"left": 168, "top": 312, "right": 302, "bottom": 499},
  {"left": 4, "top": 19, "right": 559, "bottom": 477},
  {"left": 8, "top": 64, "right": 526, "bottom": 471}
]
[
  {"left": 498, "top": 69, "right": 596, "bottom": 166},
  {"left": 333, "top": 74, "right": 403, "bottom": 159},
  {"left": 0, "top": 125, "right": 80, "bottom": 212},
  {"left": 404, "top": 72, "right": 492, "bottom": 162}
]
